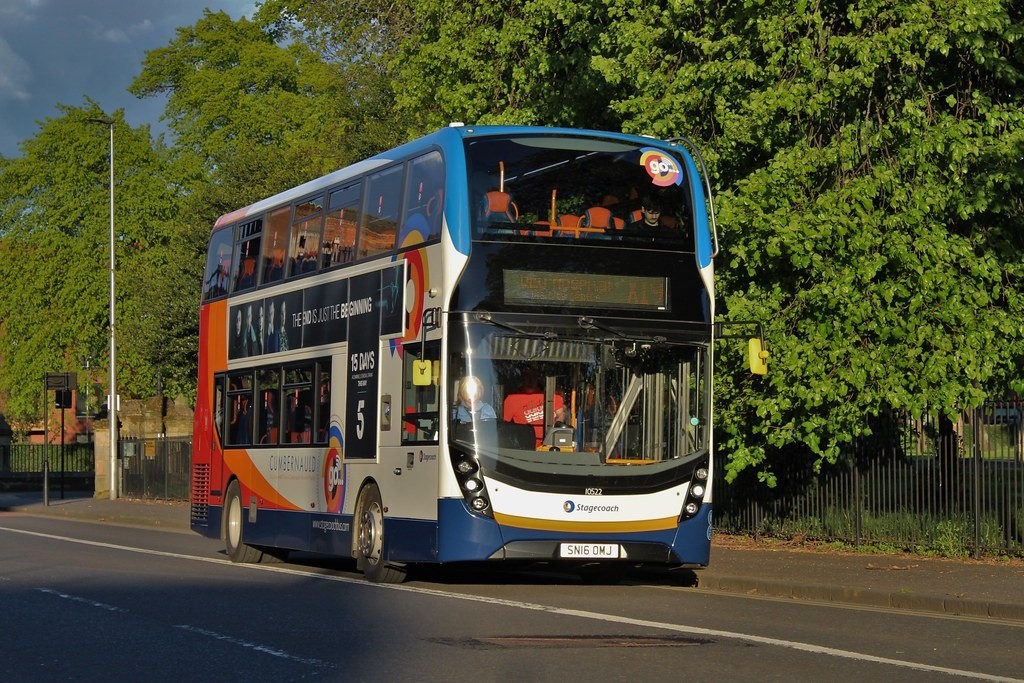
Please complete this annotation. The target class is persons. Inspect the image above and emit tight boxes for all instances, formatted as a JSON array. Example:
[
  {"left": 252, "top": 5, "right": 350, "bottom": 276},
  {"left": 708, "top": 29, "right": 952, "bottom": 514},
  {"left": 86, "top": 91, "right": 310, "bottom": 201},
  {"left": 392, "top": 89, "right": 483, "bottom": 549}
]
[
  {"left": 626, "top": 194, "right": 676, "bottom": 239},
  {"left": 451, "top": 375, "right": 497, "bottom": 422},
  {"left": 505, "top": 372, "right": 566, "bottom": 447}
]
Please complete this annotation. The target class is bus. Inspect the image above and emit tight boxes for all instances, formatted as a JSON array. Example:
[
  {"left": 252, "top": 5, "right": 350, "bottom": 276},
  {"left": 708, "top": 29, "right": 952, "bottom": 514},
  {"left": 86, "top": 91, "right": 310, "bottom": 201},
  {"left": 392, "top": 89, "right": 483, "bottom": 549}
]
[{"left": 190, "top": 122, "right": 770, "bottom": 585}]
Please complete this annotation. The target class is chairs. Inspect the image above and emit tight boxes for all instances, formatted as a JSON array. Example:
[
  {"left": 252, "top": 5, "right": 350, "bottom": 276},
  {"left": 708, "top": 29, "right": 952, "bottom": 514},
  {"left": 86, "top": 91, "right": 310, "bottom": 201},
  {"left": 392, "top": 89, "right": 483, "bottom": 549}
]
[
  {"left": 532, "top": 222, "right": 553, "bottom": 237},
  {"left": 630, "top": 210, "right": 643, "bottom": 222},
  {"left": 428, "top": 189, "right": 446, "bottom": 236},
  {"left": 611, "top": 218, "right": 626, "bottom": 240},
  {"left": 232, "top": 389, "right": 327, "bottom": 443},
  {"left": 218, "top": 249, "right": 328, "bottom": 294},
  {"left": 556, "top": 215, "right": 582, "bottom": 238},
  {"left": 482, "top": 191, "right": 520, "bottom": 235},
  {"left": 584, "top": 206, "right": 615, "bottom": 241}
]
